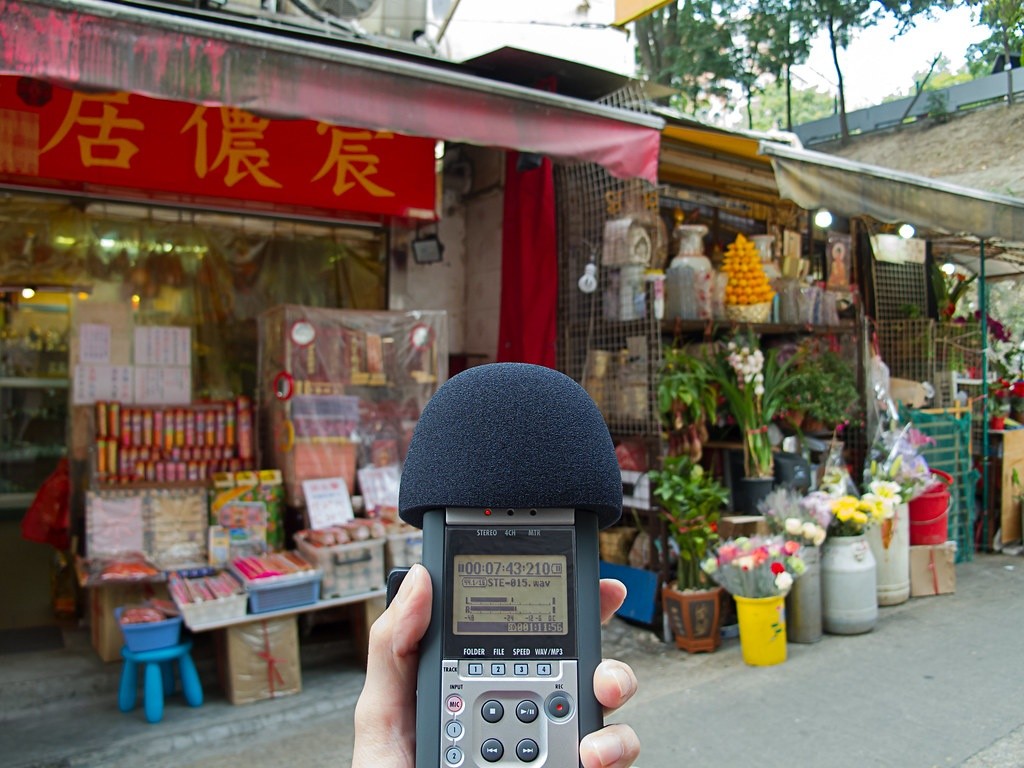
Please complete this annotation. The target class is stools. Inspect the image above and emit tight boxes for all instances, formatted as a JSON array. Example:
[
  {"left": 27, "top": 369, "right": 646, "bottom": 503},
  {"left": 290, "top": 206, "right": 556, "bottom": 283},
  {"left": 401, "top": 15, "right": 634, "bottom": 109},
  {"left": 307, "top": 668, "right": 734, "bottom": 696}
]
[{"left": 116, "top": 638, "right": 204, "bottom": 725}]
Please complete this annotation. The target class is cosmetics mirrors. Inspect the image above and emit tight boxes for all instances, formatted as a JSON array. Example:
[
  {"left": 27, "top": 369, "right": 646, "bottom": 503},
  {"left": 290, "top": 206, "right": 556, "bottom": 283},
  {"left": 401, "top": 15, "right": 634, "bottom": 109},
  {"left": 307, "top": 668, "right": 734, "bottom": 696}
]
[
  {"left": 277, "top": 420, "right": 296, "bottom": 453},
  {"left": 287, "top": 318, "right": 319, "bottom": 350},
  {"left": 273, "top": 370, "right": 296, "bottom": 402},
  {"left": 409, "top": 322, "right": 435, "bottom": 350}
]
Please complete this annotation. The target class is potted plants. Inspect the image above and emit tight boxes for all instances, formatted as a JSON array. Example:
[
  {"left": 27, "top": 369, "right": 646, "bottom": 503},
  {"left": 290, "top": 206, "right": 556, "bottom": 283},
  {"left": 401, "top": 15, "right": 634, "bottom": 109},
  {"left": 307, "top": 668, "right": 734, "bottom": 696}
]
[
  {"left": 806, "top": 351, "right": 864, "bottom": 435},
  {"left": 769, "top": 345, "right": 837, "bottom": 432},
  {"left": 647, "top": 455, "right": 733, "bottom": 653}
]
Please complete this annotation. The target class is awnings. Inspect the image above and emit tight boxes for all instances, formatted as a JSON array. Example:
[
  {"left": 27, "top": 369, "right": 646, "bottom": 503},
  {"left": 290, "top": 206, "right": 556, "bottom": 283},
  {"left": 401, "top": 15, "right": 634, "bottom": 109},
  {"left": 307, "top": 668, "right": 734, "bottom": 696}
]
[
  {"left": 1, "top": 0, "right": 665, "bottom": 187},
  {"left": 615, "top": 98, "right": 1024, "bottom": 245}
]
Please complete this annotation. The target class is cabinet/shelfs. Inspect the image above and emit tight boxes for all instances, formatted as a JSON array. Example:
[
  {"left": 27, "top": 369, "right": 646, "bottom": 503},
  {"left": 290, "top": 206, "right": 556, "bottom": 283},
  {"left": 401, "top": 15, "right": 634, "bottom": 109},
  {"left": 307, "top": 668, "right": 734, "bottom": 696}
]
[{"left": 575, "top": 317, "right": 869, "bottom": 641}]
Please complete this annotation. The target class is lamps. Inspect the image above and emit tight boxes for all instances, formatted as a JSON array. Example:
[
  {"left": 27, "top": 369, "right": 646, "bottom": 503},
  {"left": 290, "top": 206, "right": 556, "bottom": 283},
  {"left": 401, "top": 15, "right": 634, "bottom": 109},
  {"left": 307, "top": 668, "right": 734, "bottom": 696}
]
[{"left": 410, "top": 218, "right": 446, "bottom": 265}]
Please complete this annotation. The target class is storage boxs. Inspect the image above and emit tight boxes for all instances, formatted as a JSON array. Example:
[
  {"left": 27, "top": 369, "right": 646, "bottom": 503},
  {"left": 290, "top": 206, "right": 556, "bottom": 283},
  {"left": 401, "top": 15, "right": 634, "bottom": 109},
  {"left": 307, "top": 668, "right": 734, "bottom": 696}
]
[
  {"left": 113, "top": 599, "right": 183, "bottom": 653},
  {"left": 217, "top": 614, "right": 304, "bottom": 709},
  {"left": 165, "top": 576, "right": 250, "bottom": 629},
  {"left": 279, "top": 396, "right": 363, "bottom": 505},
  {"left": 90, "top": 583, "right": 170, "bottom": 664},
  {"left": 294, "top": 533, "right": 388, "bottom": 599},
  {"left": 355, "top": 596, "right": 387, "bottom": 673},
  {"left": 228, "top": 560, "right": 324, "bottom": 615},
  {"left": 385, "top": 530, "right": 424, "bottom": 569}
]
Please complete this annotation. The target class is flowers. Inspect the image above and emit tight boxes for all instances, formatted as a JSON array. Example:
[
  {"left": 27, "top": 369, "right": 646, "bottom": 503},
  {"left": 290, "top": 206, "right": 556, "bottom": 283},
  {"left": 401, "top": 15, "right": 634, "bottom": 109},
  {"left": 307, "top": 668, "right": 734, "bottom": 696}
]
[
  {"left": 982, "top": 376, "right": 1011, "bottom": 418},
  {"left": 699, "top": 532, "right": 809, "bottom": 596},
  {"left": 829, "top": 495, "right": 887, "bottom": 534},
  {"left": 859, "top": 421, "right": 935, "bottom": 521},
  {"left": 756, "top": 486, "right": 832, "bottom": 547},
  {"left": 709, "top": 328, "right": 816, "bottom": 478}
]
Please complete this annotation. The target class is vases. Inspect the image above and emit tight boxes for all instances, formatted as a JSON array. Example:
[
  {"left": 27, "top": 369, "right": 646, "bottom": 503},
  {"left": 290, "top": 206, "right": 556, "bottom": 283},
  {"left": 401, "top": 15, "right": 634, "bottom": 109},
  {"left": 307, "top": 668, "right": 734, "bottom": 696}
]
[
  {"left": 821, "top": 536, "right": 879, "bottom": 635},
  {"left": 787, "top": 545, "right": 825, "bottom": 644},
  {"left": 731, "top": 593, "right": 790, "bottom": 668},
  {"left": 990, "top": 416, "right": 1005, "bottom": 430},
  {"left": 672, "top": 224, "right": 715, "bottom": 274},
  {"left": 865, "top": 504, "right": 912, "bottom": 606},
  {"left": 751, "top": 233, "right": 781, "bottom": 278}
]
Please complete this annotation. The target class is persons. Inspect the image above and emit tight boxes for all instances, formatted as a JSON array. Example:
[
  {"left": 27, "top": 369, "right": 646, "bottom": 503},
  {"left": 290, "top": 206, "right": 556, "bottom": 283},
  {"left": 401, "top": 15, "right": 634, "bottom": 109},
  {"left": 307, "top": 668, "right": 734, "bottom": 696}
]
[{"left": 347, "top": 560, "right": 641, "bottom": 768}]
